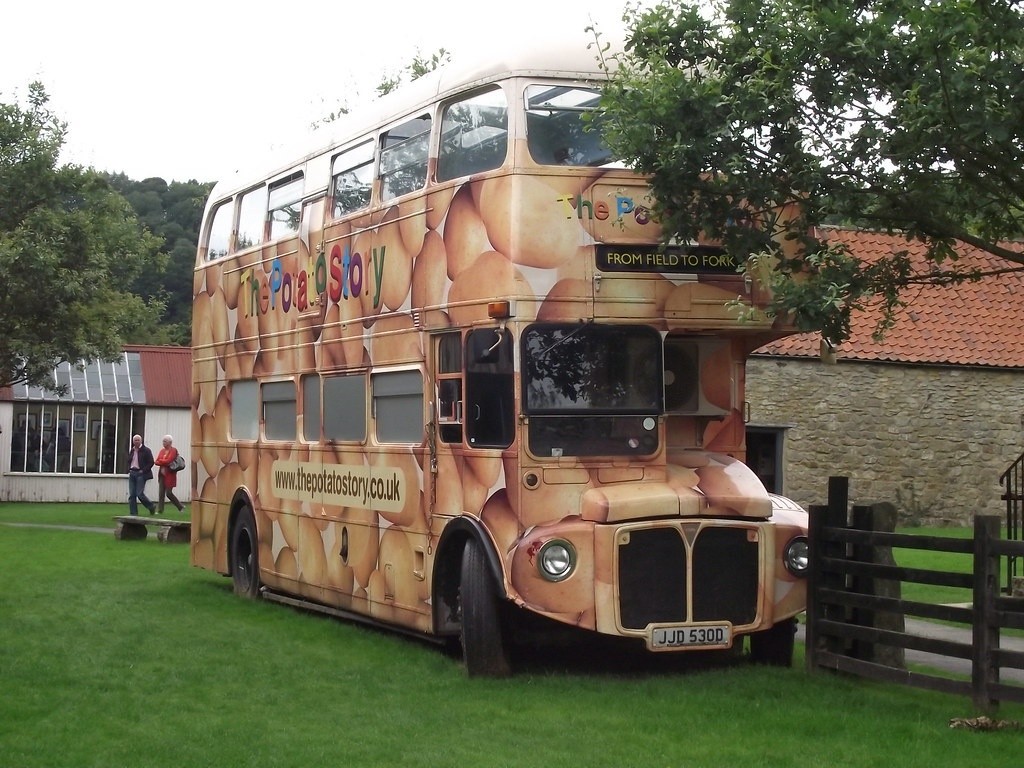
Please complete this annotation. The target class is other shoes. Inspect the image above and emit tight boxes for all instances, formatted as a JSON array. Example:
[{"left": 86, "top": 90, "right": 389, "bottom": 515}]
[
  {"left": 180, "top": 506, "right": 185, "bottom": 513},
  {"left": 150, "top": 504, "right": 156, "bottom": 516},
  {"left": 155, "top": 512, "right": 161, "bottom": 515}
]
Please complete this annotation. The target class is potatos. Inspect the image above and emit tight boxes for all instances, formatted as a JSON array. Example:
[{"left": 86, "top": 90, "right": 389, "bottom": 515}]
[{"left": 192, "top": 172, "right": 808, "bottom": 621}]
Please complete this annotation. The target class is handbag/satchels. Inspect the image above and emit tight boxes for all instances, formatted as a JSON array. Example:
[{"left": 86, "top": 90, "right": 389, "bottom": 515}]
[{"left": 169, "top": 448, "right": 186, "bottom": 471}]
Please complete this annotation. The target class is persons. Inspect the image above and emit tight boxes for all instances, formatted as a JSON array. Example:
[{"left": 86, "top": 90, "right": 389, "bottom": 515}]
[
  {"left": 154, "top": 436, "right": 186, "bottom": 514},
  {"left": 127, "top": 435, "right": 156, "bottom": 516},
  {"left": 28, "top": 425, "right": 71, "bottom": 472}
]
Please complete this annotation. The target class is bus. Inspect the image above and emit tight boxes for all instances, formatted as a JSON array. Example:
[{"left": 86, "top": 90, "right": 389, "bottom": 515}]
[{"left": 190, "top": 38, "right": 815, "bottom": 681}]
